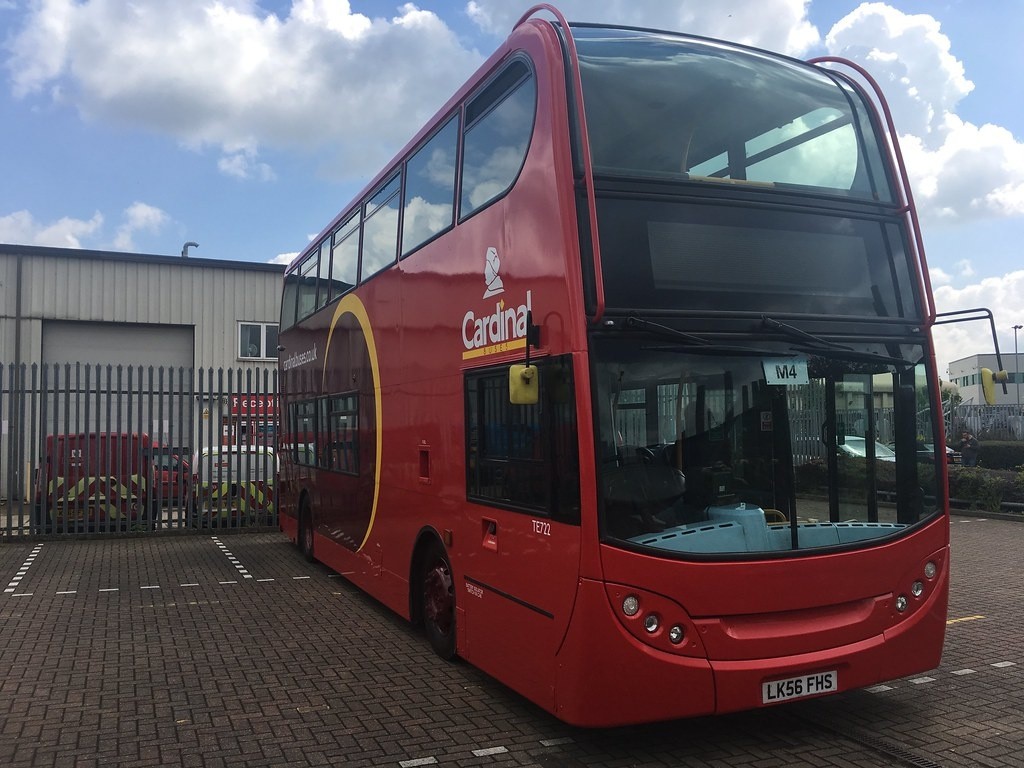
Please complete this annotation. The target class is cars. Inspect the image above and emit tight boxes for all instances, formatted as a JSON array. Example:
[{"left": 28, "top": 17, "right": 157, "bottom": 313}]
[
  {"left": 883, "top": 440, "right": 962, "bottom": 466},
  {"left": 151, "top": 441, "right": 193, "bottom": 507},
  {"left": 792, "top": 435, "right": 898, "bottom": 463}
]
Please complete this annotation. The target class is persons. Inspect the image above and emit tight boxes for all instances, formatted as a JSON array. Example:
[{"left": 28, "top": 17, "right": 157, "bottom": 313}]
[
  {"left": 956, "top": 430, "right": 980, "bottom": 467},
  {"left": 599, "top": 400, "right": 775, "bottom": 540}
]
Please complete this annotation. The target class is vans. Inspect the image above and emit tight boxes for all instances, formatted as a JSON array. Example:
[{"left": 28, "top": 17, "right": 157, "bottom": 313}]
[
  {"left": 181, "top": 443, "right": 278, "bottom": 528},
  {"left": 35, "top": 430, "right": 155, "bottom": 534}
]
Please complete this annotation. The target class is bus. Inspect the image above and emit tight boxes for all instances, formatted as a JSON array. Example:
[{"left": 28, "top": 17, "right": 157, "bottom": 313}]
[{"left": 275, "top": 4, "right": 1012, "bottom": 729}]
